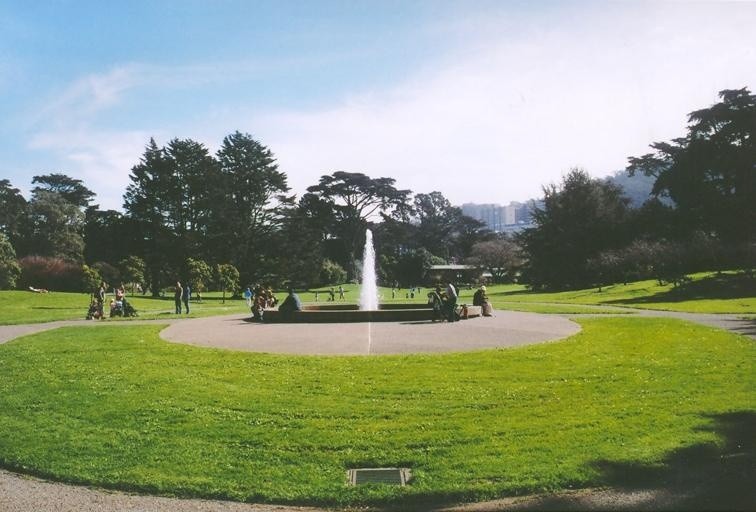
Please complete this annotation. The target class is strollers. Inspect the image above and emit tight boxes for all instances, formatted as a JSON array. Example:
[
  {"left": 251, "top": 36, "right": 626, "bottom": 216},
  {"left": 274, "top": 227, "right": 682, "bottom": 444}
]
[
  {"left": 87, "top": 305, "right": 101, "bottom": 320},
  {"left": 110, "top": 301, "right": 123, "bottom": 317},
  {"left": 427, "top": 291, "right": 460, "bottom": 322}
]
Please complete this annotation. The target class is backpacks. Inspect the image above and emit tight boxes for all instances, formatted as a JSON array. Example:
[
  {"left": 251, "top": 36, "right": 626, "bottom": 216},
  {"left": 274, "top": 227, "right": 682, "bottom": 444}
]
[{"left": 94, "top": 287, "right": 101, "bottom": 298}]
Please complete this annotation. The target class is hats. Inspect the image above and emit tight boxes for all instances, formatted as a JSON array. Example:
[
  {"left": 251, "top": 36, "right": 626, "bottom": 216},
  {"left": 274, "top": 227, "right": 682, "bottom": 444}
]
[{"left": 481, "top": 286, "right": 487, "bottom": 291}]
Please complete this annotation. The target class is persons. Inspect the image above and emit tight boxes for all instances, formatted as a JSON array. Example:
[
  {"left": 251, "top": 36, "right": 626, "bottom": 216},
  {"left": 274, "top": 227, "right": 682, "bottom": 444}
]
[
  {"left": 183, "top": 281, "right": 191, "bottom": 314},
  {"left": 196, "top": 288, "right": 202, "bottom": 303},
  {"left": 434, "top": 279, "right": 460, "bottom": 322},
  {"left": 109, "top": 280, "right": 150, "bottom": 318},
  {"left": 338, "top": 286, "right": 345, "bottom": 302},
  {"left": 328, "top": 286, "right": 335, "bottom": 301},
  {"left": 174, "top": 281, "right": 183, "bottom": 315},
  {"left": 391, "top": 288, "right": 395, "bottom": 299},
  {"left": 84, "top": 281, "right": 107, "bottom": 319},
  {"left": 470, "top": 274, "right": 492, "bottom": 317},
  {"left": 243, "top": 281, "right": 279, "bottom": 323}
]
